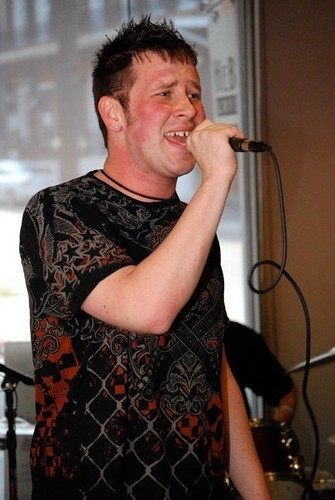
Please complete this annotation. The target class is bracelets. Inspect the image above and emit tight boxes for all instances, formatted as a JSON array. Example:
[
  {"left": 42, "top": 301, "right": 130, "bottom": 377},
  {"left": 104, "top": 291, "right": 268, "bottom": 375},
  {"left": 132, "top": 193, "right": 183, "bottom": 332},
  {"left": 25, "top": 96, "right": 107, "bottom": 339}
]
[{"left": 280, "top": 404, "right": 294, "bottom": 412}]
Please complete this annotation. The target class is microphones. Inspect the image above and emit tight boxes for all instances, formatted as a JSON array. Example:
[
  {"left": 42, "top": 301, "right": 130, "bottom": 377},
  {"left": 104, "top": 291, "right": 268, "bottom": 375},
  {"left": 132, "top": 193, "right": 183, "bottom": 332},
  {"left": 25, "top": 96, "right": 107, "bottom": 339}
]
[{"left": 229, "top": 136, "right": 272, "bottom": 153}]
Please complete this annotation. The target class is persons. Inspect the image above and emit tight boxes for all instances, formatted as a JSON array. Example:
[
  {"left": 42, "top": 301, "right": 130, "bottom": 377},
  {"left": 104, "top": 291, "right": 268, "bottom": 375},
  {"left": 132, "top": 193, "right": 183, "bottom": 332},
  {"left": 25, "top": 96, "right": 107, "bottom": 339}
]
[
  {"left": 19, "top": 13, "right": 271, "bottom": 500},
  {"left": 224, "top": 321, "right": 296, "bottom": 424}
]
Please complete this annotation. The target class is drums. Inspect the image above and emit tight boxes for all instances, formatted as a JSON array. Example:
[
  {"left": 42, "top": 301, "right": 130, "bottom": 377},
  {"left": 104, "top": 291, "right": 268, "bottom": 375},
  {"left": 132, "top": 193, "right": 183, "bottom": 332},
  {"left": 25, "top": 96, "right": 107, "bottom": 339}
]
[
  {"left": 264, "top": 471, "right": 312, "bottom": 500},
  {"left": 249, "top": 417, "right": 301, "bottom": 472}
]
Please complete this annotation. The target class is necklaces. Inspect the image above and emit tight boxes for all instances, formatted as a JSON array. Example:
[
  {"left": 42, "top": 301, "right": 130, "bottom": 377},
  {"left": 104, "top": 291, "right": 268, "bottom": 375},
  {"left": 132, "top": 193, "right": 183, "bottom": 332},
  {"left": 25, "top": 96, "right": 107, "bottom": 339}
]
[{"left": 101, "top": 169, "right": 176, "bottom": 203}]
